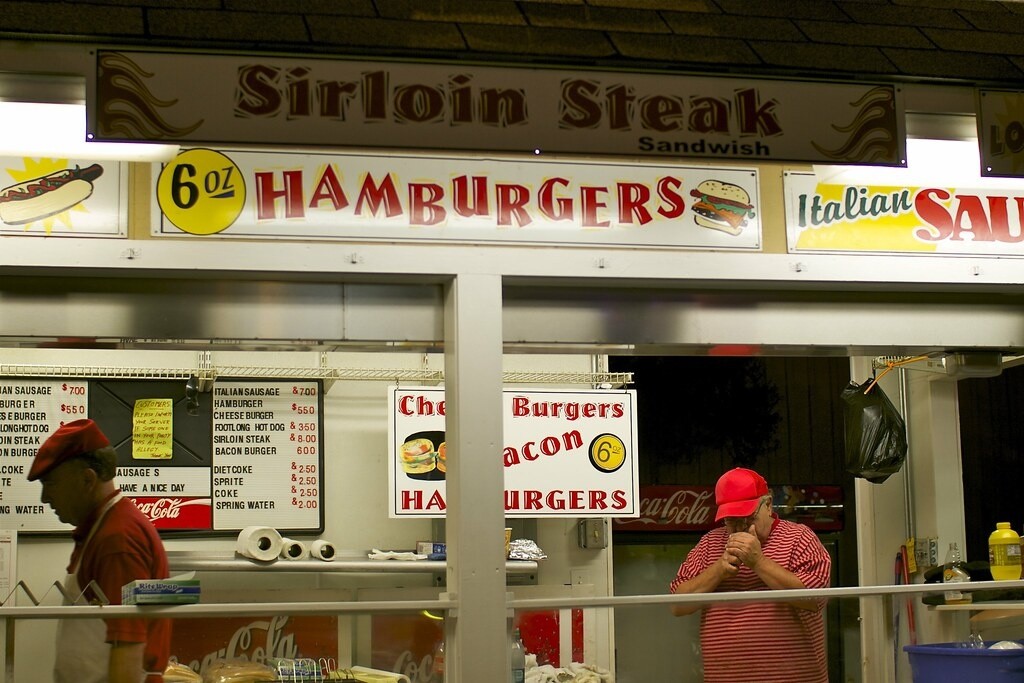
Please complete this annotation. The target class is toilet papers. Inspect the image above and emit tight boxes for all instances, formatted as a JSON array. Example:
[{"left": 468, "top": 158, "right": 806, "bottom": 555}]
[
  {"left": 235, "top": 525, "right": 283, "bottom": 561},
  {"left": 282, "top": 538, "right": 306, "bottom": 560},
  {"left": 311, "top": 540, "right": 336, "bottom": 560}
]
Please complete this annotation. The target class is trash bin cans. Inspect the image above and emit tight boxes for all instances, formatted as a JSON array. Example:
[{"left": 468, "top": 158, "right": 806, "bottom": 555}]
[{"left": 903, "top": 640, "right": 1024, "bottom": 683}]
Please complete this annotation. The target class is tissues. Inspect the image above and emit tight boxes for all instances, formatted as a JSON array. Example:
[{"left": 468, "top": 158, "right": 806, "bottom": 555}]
[{"left": 122, "top": 572, "right": 201, "bottom": 605}]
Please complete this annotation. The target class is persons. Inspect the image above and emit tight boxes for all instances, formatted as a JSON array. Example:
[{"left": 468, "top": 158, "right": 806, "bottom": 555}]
[
  {"left": 669, "top": 467, "right": 831, "bottom": 683},
  {"left": 26, "top": 418, "right": 172, "bottom": 683}
]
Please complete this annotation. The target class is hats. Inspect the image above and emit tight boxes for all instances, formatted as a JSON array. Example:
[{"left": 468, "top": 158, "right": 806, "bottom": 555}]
[
  {"left": 27, "top": 419, "right": 111, "bottom": 482},
  {"left": 714, "top": 467, "right": 769, "bottom": 521}
]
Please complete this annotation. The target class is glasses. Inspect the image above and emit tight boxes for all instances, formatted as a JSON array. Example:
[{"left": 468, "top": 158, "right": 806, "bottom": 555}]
[{"left": 725, "top": 501, "right": 765, "bottom": 524}]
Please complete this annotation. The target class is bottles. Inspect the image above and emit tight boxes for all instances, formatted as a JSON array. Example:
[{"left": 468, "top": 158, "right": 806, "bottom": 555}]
[
  {"left": 432, "top": 642, "right": 445, "bottom": 683},
  {"left": 512, "top": 631, "right": 526, "bottom": 683},
  {"left": 943, "top": 543, "right": 972, "bottom": 603},
  {"left": 989, "top": 522, "right": 1022, "bottom": 579}
]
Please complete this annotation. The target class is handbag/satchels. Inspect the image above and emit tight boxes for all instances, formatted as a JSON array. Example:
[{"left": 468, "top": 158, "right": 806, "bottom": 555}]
[{"left": 832, "top": 378, "right": 908, "bottom": 484}]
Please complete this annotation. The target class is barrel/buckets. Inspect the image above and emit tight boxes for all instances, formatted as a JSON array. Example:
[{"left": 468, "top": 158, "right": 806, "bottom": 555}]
[{"left": 505, "top": 527, "right": 512, "bottom": 559}]
